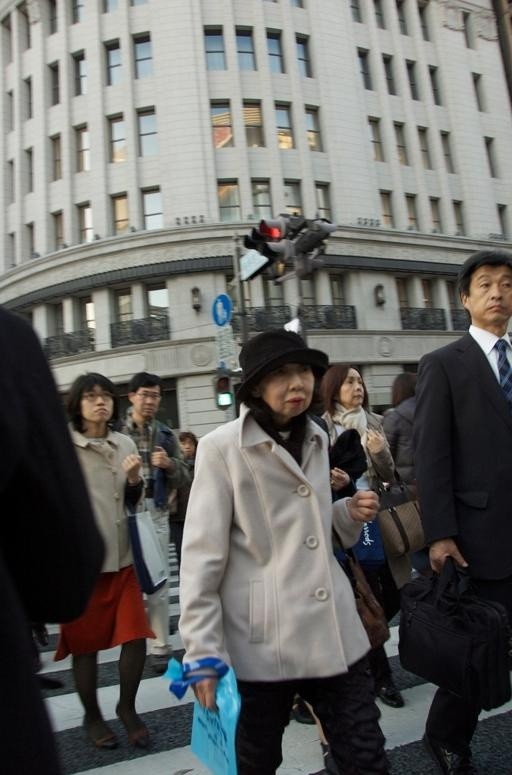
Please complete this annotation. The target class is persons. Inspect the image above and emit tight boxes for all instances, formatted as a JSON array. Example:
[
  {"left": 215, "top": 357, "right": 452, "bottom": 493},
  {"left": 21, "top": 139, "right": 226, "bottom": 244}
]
[
  {"left": 178, "top": 328, "right": 394, "bottom": 775},
  {"left": 0, "top": 306, "right": 198, "bottom": 774},
  {"left": 413, "top": 251, "right": 511, "bottom": 775},
  {"left": 291, "top": 365, "right": 424, "bottom": 725}
]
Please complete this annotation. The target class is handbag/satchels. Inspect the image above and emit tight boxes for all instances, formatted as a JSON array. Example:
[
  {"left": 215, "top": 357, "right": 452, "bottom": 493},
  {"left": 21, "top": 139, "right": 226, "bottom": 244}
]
[
  {"left": 398, "top": 559, "right": 511, "bottom": 710},
  {"left": 127, "top": 501, "right": 171, "bottom": 593},
  {"left": 344, "top": 555, "right": 391, "bottom": 648},
  {"left": 366, "top": 445, "right": 427, "bottom": 555}
]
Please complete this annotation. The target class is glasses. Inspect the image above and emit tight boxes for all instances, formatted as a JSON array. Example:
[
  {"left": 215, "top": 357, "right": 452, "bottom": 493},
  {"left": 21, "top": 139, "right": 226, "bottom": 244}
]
[
  {"left": 82, "top": 391, "right": 114, "bottom": 402},
  {"left": 134, "top": 393, "right": 164, "bottom": 400}
]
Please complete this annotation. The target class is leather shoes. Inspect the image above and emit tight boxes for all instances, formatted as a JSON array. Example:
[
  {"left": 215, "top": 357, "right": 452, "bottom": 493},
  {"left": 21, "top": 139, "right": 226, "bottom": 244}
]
[
  {"left": 84, "top": 715, "right": 120, "bottom": 749},
  {"left": 374, "top": 679, "right": 406, "bottom": 708},
  {"left": 116, "top": 704, "right": 150, "bottom": 749},
  {"left": 422, "top": 732, "right": 475, "bottom": 775},
  {"left": 292, "top": 697, "right": 316, "bottom": 723}
]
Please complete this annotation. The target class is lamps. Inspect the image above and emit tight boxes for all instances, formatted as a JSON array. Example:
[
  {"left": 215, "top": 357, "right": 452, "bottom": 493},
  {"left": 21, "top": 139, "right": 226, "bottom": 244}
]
[
  {"left": 373, "top": 282, "right": 386, "bottom": 305},
  {"left": 191, "top": 286, "right": 202, "bottom": 311}
]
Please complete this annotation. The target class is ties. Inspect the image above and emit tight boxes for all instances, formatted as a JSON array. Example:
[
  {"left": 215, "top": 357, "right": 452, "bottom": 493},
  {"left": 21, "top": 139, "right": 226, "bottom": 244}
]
[{"left": 493, "top": 339, "right": 512, "bottom": 400}]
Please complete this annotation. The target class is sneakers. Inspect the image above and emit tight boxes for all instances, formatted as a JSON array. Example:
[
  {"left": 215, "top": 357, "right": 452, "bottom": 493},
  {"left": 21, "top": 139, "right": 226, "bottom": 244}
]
[{"left": 150, "top": 653, "right": 173, "bottom": 674}]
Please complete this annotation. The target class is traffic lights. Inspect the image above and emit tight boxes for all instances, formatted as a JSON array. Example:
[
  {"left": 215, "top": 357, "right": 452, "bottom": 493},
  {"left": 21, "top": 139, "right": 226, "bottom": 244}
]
[
  {"left": 245, "top": 219, "right": 282, "bottom": 251},
  {"left": 300, "top": 221, "right": 336, "bottom": 255},
  {"left": 260, "top": 242, "right": 284, "bottom": 259},
  {"left": 306, "top": 259, "right": 323, "bottom": 278},
  {"left": 214, "top": 375, "right": 232, "bottom": 409}
]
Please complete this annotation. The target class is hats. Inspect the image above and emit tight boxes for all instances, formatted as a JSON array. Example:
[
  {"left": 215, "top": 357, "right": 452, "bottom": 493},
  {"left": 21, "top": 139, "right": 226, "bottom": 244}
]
[{"left": 236, "top": 330, "right": 328, "bottom": 399}]
